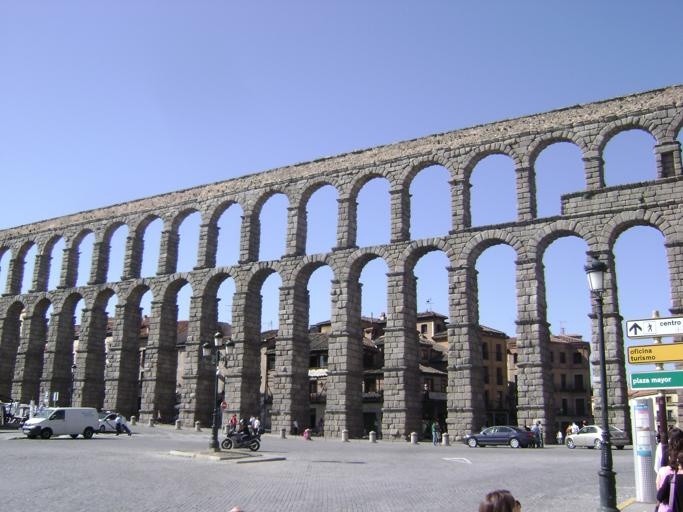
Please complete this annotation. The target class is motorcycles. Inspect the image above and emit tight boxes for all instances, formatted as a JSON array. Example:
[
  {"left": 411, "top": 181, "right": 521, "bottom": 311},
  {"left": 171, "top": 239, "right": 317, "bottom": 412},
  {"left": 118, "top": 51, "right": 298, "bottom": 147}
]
[{"left": 221, "top": 425, "right": 264, "bottom": 451}]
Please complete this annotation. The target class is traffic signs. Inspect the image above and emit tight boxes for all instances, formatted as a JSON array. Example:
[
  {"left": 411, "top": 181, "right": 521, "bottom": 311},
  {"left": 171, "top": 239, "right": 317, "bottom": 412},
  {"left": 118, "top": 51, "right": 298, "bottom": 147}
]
[
  {"left": 629, "top": 370, "right": 683, "bottom": 390},
  {"left": 627, "top": 342, "right": 683, "bottom": 364},
  {"left": 626, "top": 316, "right": 683, "bottom": 339}
]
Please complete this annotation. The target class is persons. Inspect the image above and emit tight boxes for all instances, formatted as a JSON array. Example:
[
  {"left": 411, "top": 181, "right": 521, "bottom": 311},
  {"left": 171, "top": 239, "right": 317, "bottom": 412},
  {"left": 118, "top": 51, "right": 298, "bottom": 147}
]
[
  {"left": 318, "top": 418, "right": 324, "bottom": 435},
  {"left": 520, "top": 416, "right": 588, "bottom": 448},
  {"left": 435, "top": 417, "right": 443, "bottom": 441},
  {"left": 431, "top": 421, "right": 440, "bottom": 444},
  {"left": 304, "top": 426, "right": 312, "bottom": 441},
  {"left": 653, "top": 419, "right": 682, "bottom": 512},
  {"left": 228, "top": 413, "right": 259, "bottom": 444},
  {"left": 115, "top": 412, "right": 121, "bottom": 435},
  {"left": 479, "top": 490, "right": 522, "bottom": 511}
]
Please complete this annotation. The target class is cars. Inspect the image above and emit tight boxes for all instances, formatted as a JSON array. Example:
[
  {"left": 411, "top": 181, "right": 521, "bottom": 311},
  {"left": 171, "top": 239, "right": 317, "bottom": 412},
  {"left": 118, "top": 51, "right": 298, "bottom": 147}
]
[
  {"left": 462, "top": 425, "right": 540, "bottom": 448},
  {"left": 564, "top": 424, "right": 629, "bottom": 450},
  {"left": 98, "top": 410, "right": 127, "bottom": 433}
]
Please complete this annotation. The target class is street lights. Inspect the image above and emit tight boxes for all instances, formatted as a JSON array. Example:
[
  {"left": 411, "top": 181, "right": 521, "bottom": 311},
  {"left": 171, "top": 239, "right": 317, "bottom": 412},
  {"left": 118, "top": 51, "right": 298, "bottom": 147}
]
[
  {"left": 200, "top": 327, "right": 234, "bottom": 451},
  {"left": 581, "top": 252, "right": 620, "bottom": 512}
]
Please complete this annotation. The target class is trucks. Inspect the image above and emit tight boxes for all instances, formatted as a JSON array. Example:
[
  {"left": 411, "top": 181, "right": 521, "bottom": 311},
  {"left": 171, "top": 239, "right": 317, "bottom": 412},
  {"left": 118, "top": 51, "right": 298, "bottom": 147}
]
[{"left": 22, "top": 407, "right": 99, "bottom": 440}]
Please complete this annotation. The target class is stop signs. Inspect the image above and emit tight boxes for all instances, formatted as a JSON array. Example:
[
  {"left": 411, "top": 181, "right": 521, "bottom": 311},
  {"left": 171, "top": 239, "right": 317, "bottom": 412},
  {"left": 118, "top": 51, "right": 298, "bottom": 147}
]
[{"left": 221, "top": 402, "right": 227, "bottom": 409}]
